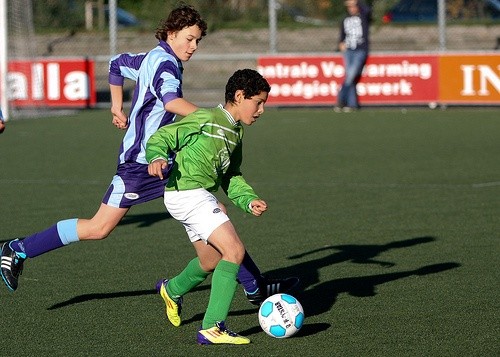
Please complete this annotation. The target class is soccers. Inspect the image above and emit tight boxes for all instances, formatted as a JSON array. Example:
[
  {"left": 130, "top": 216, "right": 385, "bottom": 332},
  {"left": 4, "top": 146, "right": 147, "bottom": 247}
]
[{"left": 258, "top": 293, "right": 305, "bottom": 339}]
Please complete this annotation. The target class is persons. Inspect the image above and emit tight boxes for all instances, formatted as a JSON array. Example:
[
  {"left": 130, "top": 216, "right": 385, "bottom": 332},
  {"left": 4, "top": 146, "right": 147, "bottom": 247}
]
[
  {"left": 0, "top": 1, "right": 299, "bottom": 307},
  {"left": 145, "top": 69, "right": 271, "bottom": 345},
  {"left": 333, "top": 0, "right": 373, "bottom": 113}
]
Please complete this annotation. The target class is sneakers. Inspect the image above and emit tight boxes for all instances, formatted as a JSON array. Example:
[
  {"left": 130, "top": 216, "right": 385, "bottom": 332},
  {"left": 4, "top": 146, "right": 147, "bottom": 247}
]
[
  {"left": 0, "top": 238, "right": 28, "bottom": 291},
  {"left": 197, "top": 320, "right": 251, "bottom": 346},
  {"left": 244, "top": 277, "right": 299, "bottom": 307},
  {"left": 155, "top": 278, "right": 183, "bottom": 327}
]
